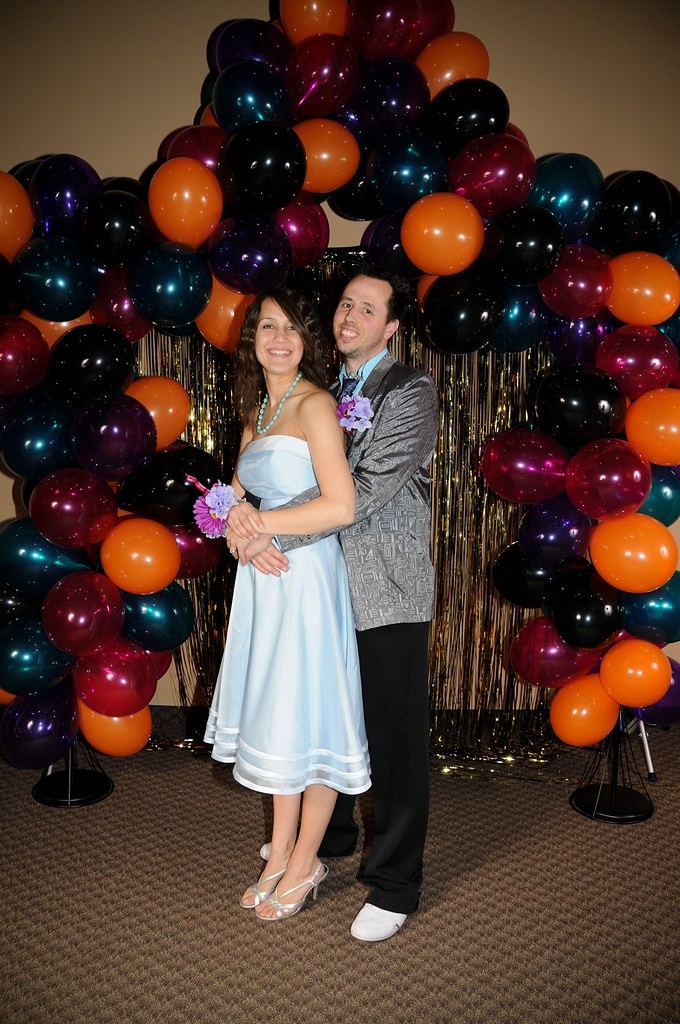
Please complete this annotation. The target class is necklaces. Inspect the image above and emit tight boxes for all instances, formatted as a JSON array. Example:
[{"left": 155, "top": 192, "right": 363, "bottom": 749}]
[{"left": 256, "top": 372, "right": 303, "bottom": 435}]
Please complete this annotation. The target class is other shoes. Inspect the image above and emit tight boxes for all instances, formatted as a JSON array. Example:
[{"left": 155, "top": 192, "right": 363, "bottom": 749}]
[
  {"left": 259, "top": 841, "right": 272, "bottom": 861},
  {"left": 350, "top": 903, "right": 409, "bottom": 942}
]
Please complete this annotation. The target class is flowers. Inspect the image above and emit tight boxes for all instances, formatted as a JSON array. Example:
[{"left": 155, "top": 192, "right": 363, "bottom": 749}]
[{"left": 336, "top": 393, "right": 374, "bottom": 436}]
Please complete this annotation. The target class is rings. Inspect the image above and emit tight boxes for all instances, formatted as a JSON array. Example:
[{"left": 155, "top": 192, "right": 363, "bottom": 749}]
[{"left": 230, "top": 548, "right": 236, "bottom": 553}]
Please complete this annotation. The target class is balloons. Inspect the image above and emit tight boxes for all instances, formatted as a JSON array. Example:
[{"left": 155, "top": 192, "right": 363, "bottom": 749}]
[{"left": 0, "top": 0, "right": 680, "bottom": 771}]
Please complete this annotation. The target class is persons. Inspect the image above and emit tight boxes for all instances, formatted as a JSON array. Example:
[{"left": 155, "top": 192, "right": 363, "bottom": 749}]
[{"left": 202, "top": 269, "right": 442, "bottom": 943}]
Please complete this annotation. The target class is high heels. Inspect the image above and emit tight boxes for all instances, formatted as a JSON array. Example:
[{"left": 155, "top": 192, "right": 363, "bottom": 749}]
[
  {"left": 255, "top": 860, "right": 329, "bottom": 921},
  {"left": 239, "top": 868, "right": 286, "bottom": 909}
]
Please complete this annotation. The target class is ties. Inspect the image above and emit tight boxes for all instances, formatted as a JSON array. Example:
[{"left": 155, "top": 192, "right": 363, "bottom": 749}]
[{"left": 337, "top": 374, "right": 359, "bottom": 405}]
